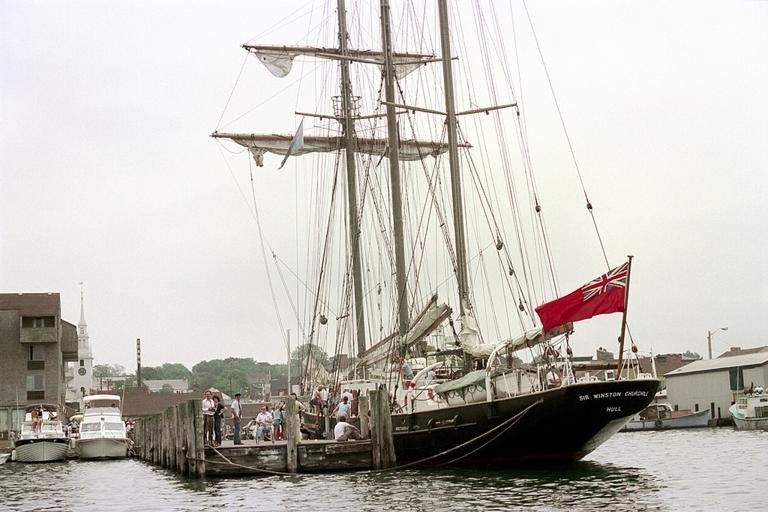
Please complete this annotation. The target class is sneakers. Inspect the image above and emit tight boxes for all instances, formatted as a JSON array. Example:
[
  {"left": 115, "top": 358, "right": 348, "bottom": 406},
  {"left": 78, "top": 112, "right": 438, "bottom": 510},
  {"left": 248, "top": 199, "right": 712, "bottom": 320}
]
[
  {"left": 234, "top": 443, "right": 245, "bottom": 445},
  {"left": 209, "top": 441, "right": 222, "bottom": 448}
]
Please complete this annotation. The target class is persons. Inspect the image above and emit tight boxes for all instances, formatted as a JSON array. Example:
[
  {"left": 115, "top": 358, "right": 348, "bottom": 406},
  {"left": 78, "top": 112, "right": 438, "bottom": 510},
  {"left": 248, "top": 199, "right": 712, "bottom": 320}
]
[
  {"left": 30, "top": 405, "right": 136, "bottom": 440},
  {"left": 201, "top": 390, "right": 216, "bottom": 447},
  {"left": 743, "top": 384, "right": 768, "bottom": 395},
  {"left": 546, "top": 363, "right": 561, "bottom": 388},
  {"left": 427, "top": 367, "right": 437, "bottom": 380},
  {"left": 385, "top": 357, "right": 414, "bottom": 379},
  {"left": 255, "top": 385, "right": 371, "bottom": 442},
  {"left": 213, "top": 395, "right": 228, "bottom": 446},
  {"left": 231, "top": 393, "right": 244, "bottom": 444}
]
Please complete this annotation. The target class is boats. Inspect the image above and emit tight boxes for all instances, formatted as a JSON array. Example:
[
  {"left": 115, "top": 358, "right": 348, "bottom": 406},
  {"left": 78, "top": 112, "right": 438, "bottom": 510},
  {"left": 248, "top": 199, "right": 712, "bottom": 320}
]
[
  {"left": 75, "top": 392, "right": 132, "bottom": 461},
  {"left": 726, "top": 384, "right": 767, "bottom": 432},
  {"left": 621, "top": 404, "right": 710, "bottom": 433},
  {"left": 10, "top": 404, "right": 72, "bottom": 462}
]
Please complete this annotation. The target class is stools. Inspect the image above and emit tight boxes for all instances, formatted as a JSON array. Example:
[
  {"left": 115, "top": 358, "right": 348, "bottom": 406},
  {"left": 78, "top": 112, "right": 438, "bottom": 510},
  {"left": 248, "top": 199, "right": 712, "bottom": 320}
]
[{"left": 255, "top": 425, "right": 274, "bottom": 444}]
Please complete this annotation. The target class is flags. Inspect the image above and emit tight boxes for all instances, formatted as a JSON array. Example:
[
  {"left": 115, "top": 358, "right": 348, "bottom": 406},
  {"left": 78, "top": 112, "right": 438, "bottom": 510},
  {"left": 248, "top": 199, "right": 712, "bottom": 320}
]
[{"left": 534, "top": 261, "right": 629, "bottom": 334}]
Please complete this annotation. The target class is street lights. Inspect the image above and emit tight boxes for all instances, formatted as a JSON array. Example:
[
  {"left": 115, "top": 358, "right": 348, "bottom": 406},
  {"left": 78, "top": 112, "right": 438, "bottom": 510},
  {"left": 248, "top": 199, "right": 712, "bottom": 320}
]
[{"left": 707, "top": 327, "right": 728, "bottom": 359}]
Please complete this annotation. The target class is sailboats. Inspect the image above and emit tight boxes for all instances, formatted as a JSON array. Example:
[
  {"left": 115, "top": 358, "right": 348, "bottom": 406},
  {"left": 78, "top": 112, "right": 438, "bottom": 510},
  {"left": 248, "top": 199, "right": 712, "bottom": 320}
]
[{"left": 206, "top": 0, "right": 665, "bottom": 477}]
